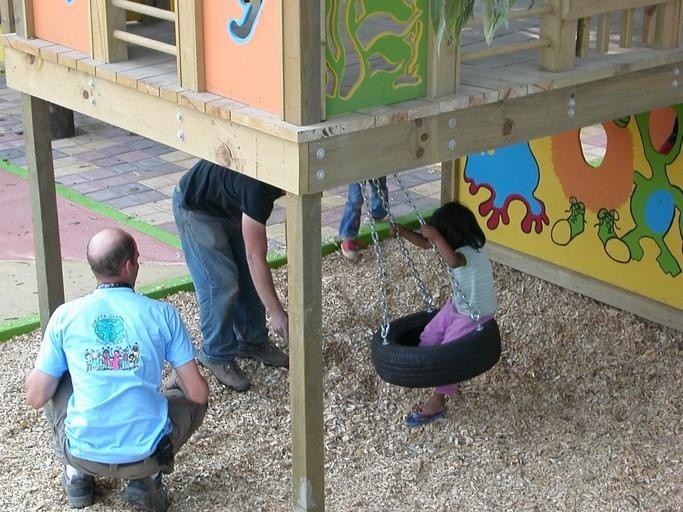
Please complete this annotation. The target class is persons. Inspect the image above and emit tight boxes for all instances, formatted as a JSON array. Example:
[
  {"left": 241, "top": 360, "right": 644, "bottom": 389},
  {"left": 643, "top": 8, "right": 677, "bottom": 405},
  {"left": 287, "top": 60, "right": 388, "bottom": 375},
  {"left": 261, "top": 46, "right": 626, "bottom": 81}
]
[
  {"left": 172, "top": 160, "right": 289, "bottom": 392},
  {"left": 340, "top": 176, "right": 395, "bottom": 261},
  {"left": 25, "top": 228, "right": 208, "bottom": 512},
  {"left": 391, "top": 202, "right": 496, "bottom": 426}
]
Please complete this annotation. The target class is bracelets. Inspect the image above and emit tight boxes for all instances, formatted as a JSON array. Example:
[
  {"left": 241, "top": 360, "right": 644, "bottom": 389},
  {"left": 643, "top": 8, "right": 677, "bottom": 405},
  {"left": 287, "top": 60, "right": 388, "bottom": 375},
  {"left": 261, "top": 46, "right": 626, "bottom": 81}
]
[{"left": 174, "top": 376, "right": 178, "bottom": 388}]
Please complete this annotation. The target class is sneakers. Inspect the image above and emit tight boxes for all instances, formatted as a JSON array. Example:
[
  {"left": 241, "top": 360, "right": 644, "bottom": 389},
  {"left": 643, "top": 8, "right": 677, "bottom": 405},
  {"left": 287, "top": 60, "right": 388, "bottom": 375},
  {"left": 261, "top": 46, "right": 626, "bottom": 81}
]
[
  {"left": 63, "top": 464, "right": 95, "bottom": 507},
  {"left": 341, "top": 240, "right": 357, "bottom": 260},
  {"left": 125, "top": 471, "right": 166, "bottom": 512}
]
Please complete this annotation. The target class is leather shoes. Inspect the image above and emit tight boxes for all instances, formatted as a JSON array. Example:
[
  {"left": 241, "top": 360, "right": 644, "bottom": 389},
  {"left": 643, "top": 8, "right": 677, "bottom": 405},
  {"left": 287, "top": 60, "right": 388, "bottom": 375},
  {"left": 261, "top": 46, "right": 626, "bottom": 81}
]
[
  {"left": 197, "top": 349, "right": 251, "bottom": 391},
  {"left": 236, "top": 343, "right": 289, "bottom": 367}
]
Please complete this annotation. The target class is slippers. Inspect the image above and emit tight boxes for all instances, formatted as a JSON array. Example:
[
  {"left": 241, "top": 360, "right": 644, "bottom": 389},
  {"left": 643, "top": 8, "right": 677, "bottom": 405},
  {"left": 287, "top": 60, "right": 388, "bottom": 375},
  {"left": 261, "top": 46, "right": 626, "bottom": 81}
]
[{"left": 405, "top": 408, "right": 445, "bottom": 426}]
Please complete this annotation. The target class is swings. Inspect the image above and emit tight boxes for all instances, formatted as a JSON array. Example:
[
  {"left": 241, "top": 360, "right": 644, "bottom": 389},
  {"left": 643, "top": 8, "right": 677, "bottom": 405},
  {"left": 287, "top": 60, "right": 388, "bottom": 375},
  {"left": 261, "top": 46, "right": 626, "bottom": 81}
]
[{"left": 358, "top": 174, "right": 500, "bottom": 386}]
